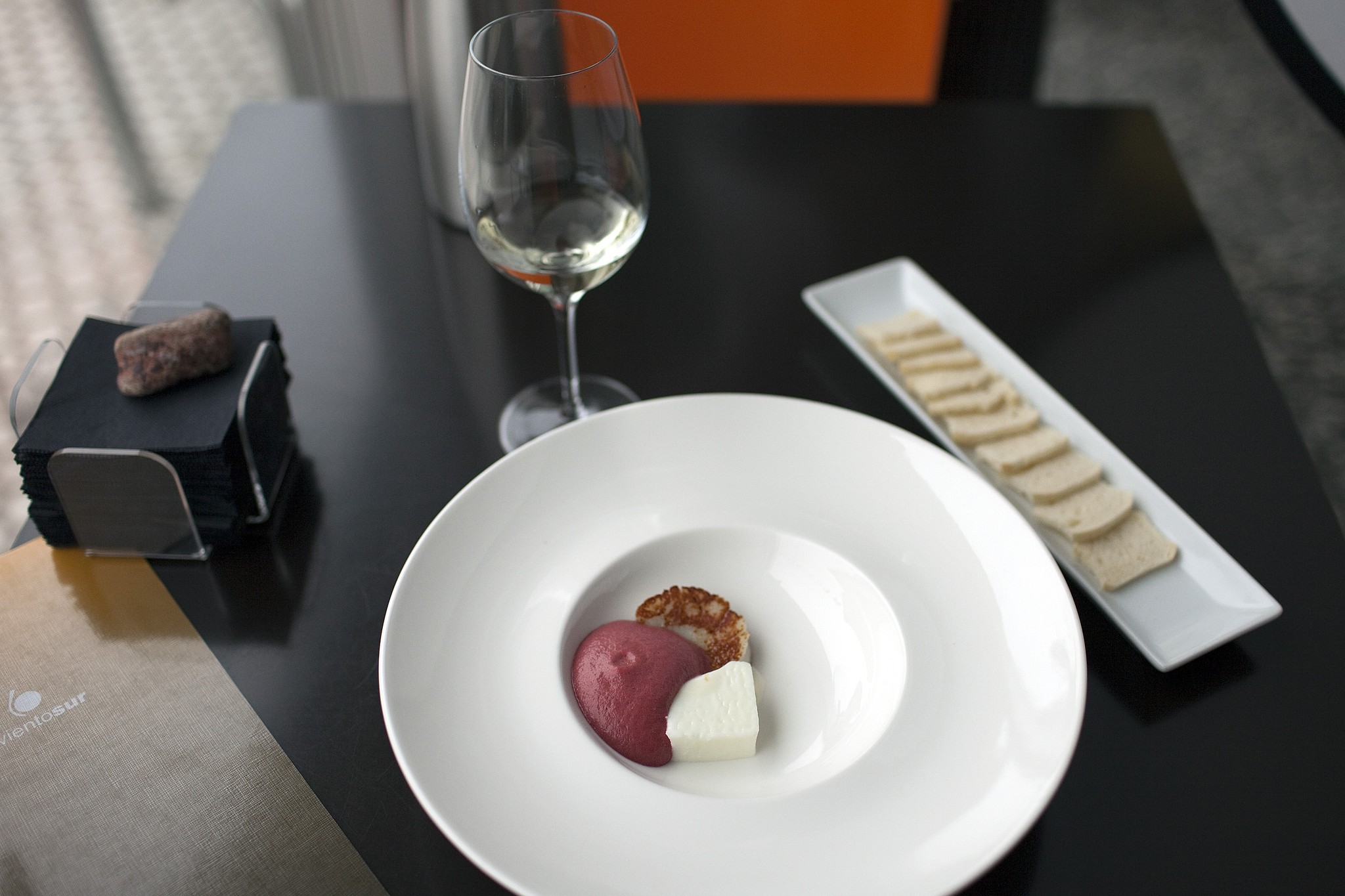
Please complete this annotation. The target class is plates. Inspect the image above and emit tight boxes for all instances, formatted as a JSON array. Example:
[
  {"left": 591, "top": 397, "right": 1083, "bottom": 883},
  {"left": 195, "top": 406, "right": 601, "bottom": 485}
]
[
  {"left": 378, "top": 393, "right": 1088, "bottom": 896},
  {"left": 802, "top": 256, "right": 1282, "bottom": 671}
]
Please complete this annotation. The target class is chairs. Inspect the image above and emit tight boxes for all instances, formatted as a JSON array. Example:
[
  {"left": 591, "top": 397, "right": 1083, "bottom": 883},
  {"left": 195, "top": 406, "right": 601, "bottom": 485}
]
[{"left": 466, "top": 0, "right": 1057, "bottom": 106}]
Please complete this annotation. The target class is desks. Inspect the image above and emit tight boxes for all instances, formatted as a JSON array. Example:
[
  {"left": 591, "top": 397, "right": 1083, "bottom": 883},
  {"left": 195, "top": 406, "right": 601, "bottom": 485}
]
[{"left": 0, "top": 88, "right": 1345, "bottom": 896}]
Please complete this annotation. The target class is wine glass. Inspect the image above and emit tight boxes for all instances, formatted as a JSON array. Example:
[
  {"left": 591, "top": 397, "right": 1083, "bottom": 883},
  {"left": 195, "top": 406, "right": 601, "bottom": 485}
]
[{"left": 458, "top": 11, "right": 647, "bottom": 451}]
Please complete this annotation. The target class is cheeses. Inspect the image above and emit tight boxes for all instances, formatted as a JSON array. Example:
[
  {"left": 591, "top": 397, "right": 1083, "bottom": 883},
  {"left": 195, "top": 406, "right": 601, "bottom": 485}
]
[{"left": 859, "top": 312, "right": 1177, "bottom": 585}]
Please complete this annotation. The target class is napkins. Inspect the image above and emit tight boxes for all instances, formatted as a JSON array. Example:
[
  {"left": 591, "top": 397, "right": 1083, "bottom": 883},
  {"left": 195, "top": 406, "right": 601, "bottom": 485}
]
[{"left": 9, "top": 314, "right": 291, "bottom": 552}]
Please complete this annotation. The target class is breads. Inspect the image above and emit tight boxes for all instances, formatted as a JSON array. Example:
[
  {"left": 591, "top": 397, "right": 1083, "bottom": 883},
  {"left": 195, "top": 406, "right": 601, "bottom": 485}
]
[{"left": 114, "top": 309, "right": 239, "bottom": 396}]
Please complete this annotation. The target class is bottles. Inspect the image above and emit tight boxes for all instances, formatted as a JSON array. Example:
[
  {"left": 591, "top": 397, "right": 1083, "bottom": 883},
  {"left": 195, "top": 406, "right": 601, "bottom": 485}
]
[{"left": 401, "top": 2, "right": 577, "bottom": 227}]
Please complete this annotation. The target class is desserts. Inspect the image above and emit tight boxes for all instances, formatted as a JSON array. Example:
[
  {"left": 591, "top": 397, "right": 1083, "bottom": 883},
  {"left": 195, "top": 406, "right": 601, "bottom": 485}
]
[{"left": 573, "top": 586, "right": 760, "bottom": 767}]
